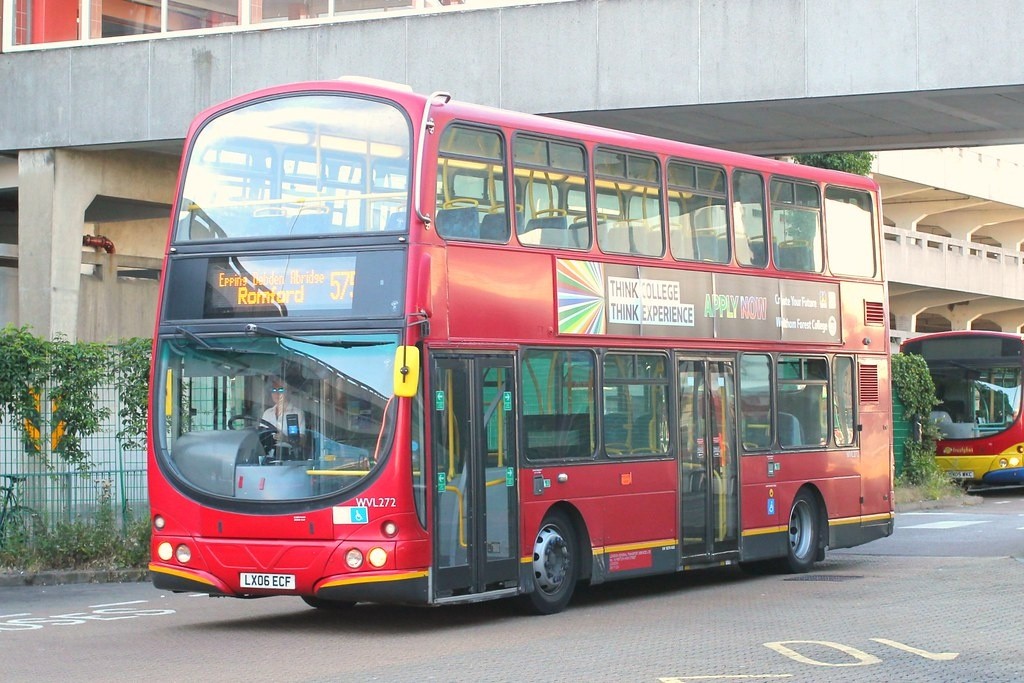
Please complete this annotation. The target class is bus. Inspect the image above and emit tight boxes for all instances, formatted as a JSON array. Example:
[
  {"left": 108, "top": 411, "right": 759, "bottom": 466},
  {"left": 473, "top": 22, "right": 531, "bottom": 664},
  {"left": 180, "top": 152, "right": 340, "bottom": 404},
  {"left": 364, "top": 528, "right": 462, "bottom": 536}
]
[
  {"left": 148, "top": 76, "right": 896, "bottom": 616},
  {"left": 898, "top": 329, "right": 1024, "bottom": 494}
]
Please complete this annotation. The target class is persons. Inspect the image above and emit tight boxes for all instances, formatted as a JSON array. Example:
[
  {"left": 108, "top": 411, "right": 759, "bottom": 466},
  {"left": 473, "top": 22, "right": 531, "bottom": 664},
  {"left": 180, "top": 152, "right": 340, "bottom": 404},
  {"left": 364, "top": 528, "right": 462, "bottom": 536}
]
[{"left": 262, "top": 379, "right": 307, "bottom": 464}]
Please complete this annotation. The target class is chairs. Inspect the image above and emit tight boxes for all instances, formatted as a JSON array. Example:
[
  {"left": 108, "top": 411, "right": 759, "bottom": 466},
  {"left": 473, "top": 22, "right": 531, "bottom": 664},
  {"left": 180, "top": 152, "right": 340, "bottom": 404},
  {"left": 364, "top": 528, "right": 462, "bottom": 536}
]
[
  {"left": 214, "top": 197, "right": 815, "bottom": 272},
  {"left": 936, "top": 400, "right": 966, "bottom": 423},
  {"left": 601, "top": 399, "right": 809, "bottom": 454}
]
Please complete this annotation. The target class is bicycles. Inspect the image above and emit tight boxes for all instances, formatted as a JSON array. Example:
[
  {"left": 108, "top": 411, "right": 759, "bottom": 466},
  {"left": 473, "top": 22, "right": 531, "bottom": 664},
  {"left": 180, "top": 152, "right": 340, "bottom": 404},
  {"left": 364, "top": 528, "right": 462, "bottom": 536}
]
[{"left": 0, "top": 475, "right": 47, "bottom": 555}]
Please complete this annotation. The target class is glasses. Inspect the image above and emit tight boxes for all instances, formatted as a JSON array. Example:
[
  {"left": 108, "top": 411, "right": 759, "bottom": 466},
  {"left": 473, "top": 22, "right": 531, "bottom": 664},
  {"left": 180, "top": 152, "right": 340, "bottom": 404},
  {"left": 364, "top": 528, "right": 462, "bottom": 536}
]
[{"left": 271, "top": 388, "right": 285, "bottom": 393}]
